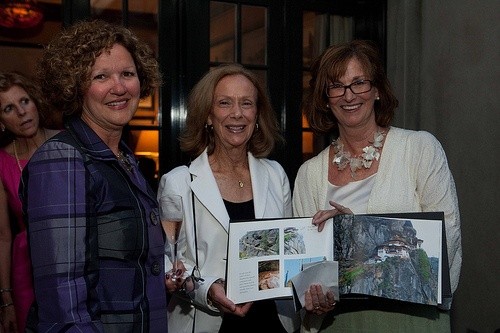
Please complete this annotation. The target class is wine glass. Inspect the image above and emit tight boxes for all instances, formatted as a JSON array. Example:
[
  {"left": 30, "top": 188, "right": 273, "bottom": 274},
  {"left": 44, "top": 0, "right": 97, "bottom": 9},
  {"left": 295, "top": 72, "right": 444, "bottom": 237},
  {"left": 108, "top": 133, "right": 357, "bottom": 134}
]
[{"left": 159, "top": 195, "right": 183, "bottom": 282}]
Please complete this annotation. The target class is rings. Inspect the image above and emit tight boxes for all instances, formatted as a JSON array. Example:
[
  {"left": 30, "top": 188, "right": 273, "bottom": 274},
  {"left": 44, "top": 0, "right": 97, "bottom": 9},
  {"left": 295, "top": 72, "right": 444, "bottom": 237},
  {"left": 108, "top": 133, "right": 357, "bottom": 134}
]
[
  {"left": 340, "top": 206, "right": 344, "bottom": 211},
  {"left": 314, "top": 306, "right": 320, "bottom": 309},
  {"left": 328, "top": 302, "right": 336, "bottom": 306}
]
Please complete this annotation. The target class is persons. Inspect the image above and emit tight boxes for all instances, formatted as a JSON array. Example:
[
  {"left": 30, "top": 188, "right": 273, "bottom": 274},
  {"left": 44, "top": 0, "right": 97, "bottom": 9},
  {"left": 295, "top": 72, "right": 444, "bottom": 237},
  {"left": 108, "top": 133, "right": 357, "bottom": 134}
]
[
  {"left": 0, "top": 71, "right": 62, "bottom": 333},
  {"left": 292, "top": 39, "right": 463, "bottom": 333},
  {"left": 17, "top": 18, "right": 194, "bottom": 333},
  {"left": 156, "top": 64, "right": 338, "bottom": 333}
]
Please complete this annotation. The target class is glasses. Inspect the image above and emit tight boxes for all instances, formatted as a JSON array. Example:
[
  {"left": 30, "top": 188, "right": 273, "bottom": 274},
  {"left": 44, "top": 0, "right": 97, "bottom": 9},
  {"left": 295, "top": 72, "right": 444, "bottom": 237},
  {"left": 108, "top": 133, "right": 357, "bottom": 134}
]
[
  {"left": 179, "top": 266, "right": 205, "bottom": 302},
  {"left": 326, "top": 80, "right": 374, "bottom": 97}
]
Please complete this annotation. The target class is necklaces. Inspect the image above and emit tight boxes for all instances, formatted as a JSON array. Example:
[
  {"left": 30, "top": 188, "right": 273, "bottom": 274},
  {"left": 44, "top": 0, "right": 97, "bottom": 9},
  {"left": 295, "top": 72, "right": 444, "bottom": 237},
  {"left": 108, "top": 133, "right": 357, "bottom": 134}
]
[
  {"left": 332, "top": 131, "right": 383, "bottom": 172},
  {"left": 117, "top": 152, "right": 122, "bottom": 156},
  {"left": 234, "top": 177, "right": 244, "bottom": 187},
  {"left": 13, "top": 128, "right": 48, "bottom": 172}
]
